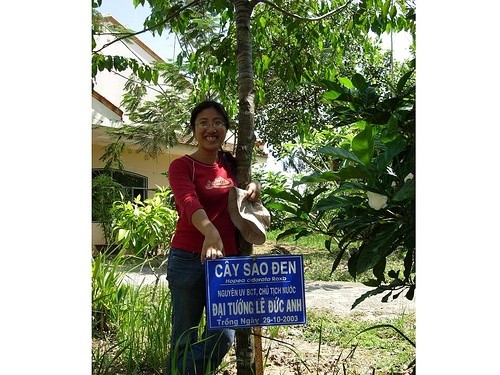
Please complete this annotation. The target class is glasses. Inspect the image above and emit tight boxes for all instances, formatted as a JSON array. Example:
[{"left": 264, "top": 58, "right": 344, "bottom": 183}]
[{"left": 195, "top": 121, "right": 225, "bottom": 128}]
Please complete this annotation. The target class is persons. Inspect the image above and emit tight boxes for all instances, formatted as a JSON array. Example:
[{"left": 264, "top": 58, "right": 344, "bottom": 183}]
[{"left": 164, "top": 99, "right": 263, "bottom": 375}]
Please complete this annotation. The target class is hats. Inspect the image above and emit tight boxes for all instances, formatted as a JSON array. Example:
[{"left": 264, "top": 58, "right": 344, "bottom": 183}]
[{"left": 228, "top": 186, "right": 270, "bottom": 245}]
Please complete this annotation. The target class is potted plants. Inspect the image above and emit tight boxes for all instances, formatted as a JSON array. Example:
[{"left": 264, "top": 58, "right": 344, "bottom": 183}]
[{"left": 92, "top": 173, "right": 133, "bottom": 255}]
